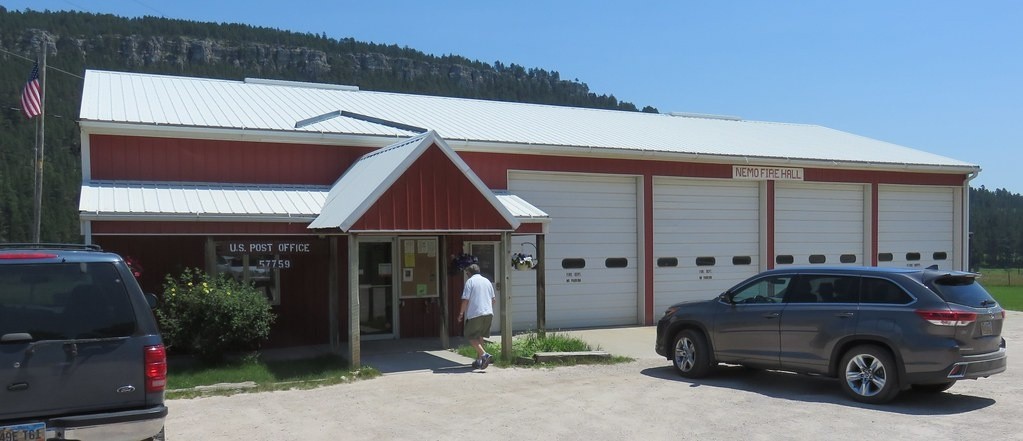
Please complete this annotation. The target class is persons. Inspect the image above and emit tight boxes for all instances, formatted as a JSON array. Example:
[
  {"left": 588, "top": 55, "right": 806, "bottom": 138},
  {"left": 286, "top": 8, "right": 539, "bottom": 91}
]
[{"left": 457, "top": 264, "right": 497, "bottom": 370}]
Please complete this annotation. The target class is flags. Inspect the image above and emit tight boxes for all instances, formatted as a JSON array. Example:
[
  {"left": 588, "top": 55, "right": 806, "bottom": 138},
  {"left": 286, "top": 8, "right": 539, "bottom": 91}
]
[{"left": 18, "top": 58, "right": 42, "bottom": 119}]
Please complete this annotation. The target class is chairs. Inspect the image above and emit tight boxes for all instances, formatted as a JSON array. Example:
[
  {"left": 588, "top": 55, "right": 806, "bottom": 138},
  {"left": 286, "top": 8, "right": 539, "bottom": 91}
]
[
  {"left": 792, "top": 278, "right": 818, "bottom": 303},
  {"left": 65, "top": 283, "right": 110, "bottom": 322}
]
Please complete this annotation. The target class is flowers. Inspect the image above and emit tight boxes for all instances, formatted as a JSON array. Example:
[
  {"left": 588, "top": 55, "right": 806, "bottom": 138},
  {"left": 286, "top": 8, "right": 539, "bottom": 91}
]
[{"left": 512, "top": 252, "right": 539, "bottom": 269}]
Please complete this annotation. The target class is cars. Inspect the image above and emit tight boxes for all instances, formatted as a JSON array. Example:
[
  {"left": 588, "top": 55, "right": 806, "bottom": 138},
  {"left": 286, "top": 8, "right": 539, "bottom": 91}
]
[{"left": 655, "top": 267, "right": 1008, "bottom": 405}]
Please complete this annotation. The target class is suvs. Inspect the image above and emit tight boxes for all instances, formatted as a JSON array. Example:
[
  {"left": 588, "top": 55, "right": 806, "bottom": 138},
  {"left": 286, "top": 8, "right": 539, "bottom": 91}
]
[{"left": 0, "top": 240, "right": 168, "bottom": 441}]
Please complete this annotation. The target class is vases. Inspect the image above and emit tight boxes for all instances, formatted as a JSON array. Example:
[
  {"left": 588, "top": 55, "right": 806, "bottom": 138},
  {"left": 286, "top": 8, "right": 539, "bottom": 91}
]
[{"left": 514, "top": 262, "right": 531, "bottom": 271}]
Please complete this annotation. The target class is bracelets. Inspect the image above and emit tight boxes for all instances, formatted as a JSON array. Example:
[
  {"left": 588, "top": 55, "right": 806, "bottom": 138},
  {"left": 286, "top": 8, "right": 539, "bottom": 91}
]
[{"left": 459, "top": 312, "right": 464, "bottom": 316}]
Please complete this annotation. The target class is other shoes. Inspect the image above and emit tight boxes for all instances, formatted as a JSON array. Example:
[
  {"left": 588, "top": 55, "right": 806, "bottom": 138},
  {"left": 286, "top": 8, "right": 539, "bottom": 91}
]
[
  {"left": 472, "top": 360, "right": 481, "bottom": 370},
  {"left": 480, "top": 353, "right": 493, "bottom": 370}
]
